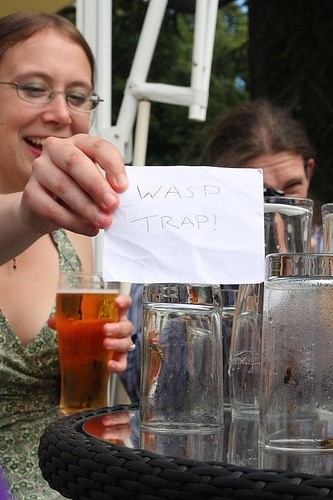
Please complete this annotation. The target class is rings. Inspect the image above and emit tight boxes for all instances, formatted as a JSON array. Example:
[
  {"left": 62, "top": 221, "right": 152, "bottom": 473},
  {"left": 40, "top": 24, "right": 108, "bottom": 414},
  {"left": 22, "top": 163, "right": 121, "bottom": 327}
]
[{"left": 127, "top": 341, "right": 136, "bottom": 353}]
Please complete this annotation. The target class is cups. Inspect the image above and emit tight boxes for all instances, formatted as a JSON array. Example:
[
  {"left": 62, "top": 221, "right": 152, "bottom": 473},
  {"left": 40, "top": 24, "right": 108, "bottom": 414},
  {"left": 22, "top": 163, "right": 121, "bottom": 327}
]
[
  {"left": 322, "top": 203, "right": 333, "bottom": 253},
  {"left": 259, "top": 253, "right": 333, "bottom": 453},
  {"left": 141, "top": 284, "right": 225, "bottom": 435},
  {"left": 56, "top": 271, "right": 121, "bottom": 414},
  {"left": 229, "top": 196, "right": 313, "bottom": 418}
]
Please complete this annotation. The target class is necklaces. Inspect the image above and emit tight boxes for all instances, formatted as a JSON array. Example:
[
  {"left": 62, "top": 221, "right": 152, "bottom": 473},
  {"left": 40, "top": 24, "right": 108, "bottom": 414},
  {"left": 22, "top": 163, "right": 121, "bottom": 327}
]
[{"left": 12, "top": 258, "right": 17, "bottom": 270}]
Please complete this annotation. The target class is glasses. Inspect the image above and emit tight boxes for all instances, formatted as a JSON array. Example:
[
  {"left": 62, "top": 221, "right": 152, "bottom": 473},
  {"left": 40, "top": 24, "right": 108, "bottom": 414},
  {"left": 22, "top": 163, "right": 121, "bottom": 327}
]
[{"left": 0, "top": 79, "right": 105, "bottom": 113}]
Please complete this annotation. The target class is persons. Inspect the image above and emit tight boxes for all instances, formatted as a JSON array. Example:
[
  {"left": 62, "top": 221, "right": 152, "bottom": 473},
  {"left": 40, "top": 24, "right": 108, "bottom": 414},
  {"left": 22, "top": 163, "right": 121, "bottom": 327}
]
[
  {"left": 1, "top": 12, "right": 134, "bottom": 500},
  {"left": 200, "top": 99, "right": 325, "bottom": 253}
]
